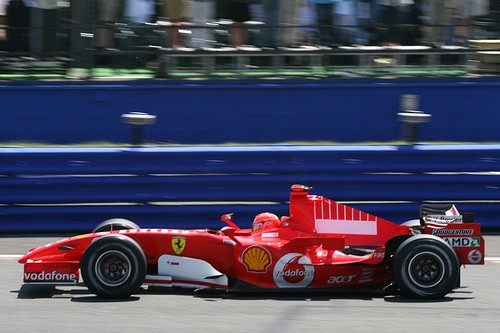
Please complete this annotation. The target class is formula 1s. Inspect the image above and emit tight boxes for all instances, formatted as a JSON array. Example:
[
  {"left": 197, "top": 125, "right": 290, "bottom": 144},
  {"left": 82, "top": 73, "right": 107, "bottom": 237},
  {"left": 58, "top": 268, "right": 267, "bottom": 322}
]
[{"left": 17, "top": 183, "right": 488, "bottom": 301}]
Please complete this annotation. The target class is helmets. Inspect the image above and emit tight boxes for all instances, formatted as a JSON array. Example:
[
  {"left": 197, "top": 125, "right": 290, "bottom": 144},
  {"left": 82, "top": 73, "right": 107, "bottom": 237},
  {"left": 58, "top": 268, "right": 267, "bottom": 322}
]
[{"left": 252, "top": 212, "right": 281, "bottom": 237}]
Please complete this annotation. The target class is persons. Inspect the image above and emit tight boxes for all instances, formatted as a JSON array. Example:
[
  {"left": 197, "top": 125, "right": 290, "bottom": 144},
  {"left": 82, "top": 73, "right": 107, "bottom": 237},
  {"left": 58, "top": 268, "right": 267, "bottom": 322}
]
[
  {"left": 0, "top": 0, "right": 500, "bottom": 70},
  {"left": 253, "top": 212, "right": 280, "bottom": 233}
]
